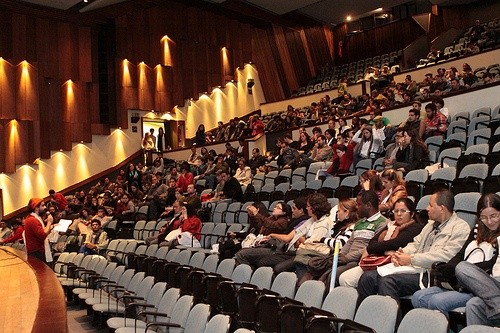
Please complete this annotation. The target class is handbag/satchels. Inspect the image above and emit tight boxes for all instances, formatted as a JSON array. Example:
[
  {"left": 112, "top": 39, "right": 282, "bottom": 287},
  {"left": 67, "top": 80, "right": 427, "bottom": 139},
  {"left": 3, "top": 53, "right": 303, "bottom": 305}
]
[
  {"left": 208, "top": 231, "right": 305, "bottom": 264},
  {"left": 433, "top": 247, "right": 496, "bottom": 294},
  {"left": 177, "top": 231, "right": 202, "bottom": 247},
  {"left": 358, "top": 253, "right": 392, "bottom": 271},
  {"left": 276, "top": 148, "right": 294, "bottom": 166},
  {"left": 293, "top": 241, "right": 331, "bottom": 266},
  {"left": 306, "top": 252, "right": 345, "bottom": 279},
  {"left": 376, "top": 262, "right": 433, "bottom": 290}
]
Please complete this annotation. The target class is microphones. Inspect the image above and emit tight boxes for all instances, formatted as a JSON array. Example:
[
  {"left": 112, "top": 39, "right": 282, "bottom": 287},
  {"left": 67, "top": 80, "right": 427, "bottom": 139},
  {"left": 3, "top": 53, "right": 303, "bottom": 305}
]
[{"left": 46, "top": 211, "right": 53, "bottom": 225}]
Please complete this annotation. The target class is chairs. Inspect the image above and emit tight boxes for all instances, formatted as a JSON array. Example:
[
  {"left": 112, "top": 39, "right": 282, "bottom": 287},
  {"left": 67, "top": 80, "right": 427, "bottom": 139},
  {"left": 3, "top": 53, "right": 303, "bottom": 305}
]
[{"left": 3, "top": 20, "right": 500, "bottom": 333}]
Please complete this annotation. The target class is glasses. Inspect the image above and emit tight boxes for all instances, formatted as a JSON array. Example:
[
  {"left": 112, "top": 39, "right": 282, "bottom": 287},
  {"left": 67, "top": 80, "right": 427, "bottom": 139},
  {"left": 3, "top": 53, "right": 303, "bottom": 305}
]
[
  {"left": 395, "top": 135, "right": 404, "bottom": 137},
  {"left": 478, "top": 211, "right": 500, "bottom": 221},
  {"left": 391, "top": 208, "right": 411, "bottom": 214}
]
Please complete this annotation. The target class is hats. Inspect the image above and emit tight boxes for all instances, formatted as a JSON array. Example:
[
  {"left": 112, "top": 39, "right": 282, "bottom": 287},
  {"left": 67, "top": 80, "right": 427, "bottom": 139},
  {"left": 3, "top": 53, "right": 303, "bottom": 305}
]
[
  {"left": 373, "top": 115, "right": 384, "bottom": 121},
  {"left": 27, "top": 197, "right": 44, "bottom": 212}
]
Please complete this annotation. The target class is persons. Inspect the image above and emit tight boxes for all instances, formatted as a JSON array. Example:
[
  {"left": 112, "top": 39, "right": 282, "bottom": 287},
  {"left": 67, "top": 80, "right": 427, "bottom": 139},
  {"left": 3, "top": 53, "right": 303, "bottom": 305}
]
[
  {"left": 1, "top": 63, "right": 499, "bottom": 332},
  {"left": 417, "top": 17, "right": 500, "bottom": 66}
]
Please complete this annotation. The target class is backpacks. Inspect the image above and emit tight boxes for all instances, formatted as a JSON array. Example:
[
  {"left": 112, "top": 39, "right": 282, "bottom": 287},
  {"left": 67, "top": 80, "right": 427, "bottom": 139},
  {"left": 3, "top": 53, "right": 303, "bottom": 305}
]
[{"left": 197, "top": 202, "right": 212, "bottom": 222}]
